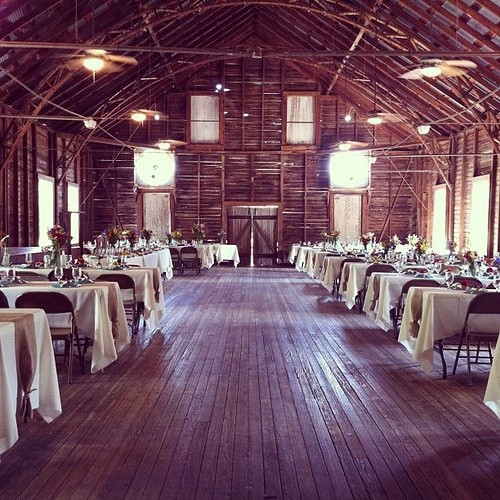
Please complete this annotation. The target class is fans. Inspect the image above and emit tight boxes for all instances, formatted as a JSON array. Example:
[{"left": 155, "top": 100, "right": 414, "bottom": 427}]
[
  {"left": 350, "top": 0, "right": 408, "bottom": 124},
  {"left": 129, "top": 51, "right": 170, "bottom": 120},
  {"left": 151, "top": 92, "right": 189, "bottom": 148},
  {"left": 47, "top": 0, "right": 138, "bottom": 74},
  {"left": 319, "top": 56, "right": 370, "bottom": 155},
  {"left": 396, "top": 0, "right": 477, "bottom": 79}
]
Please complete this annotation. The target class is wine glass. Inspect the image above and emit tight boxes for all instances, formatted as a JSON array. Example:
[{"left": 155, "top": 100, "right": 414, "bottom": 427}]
[
  {"left": 165, "top": 238, "right": 204, "bottom": 246},
  {"left": 292, "top": 238, "right": 500, "bottom": 293},
  {"left": 0, "top": 267, "right": 16, "bottom": 286},
  {"left": 66, "top": 254, "right": 72, "bottom": 268},
  {"left": 72, "top": 267, "right": 82, "bottom": 286},
  {"left": 81, "top": 239, "right": 163, "bottom": 269},
  {"left": 54, "top": 266, "right": 63, "bottom": 288}
]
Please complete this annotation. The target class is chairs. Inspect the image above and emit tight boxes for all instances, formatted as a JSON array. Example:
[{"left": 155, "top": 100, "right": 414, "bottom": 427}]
[
  {"left": 485, "top": 279, "right": 500, "bottom": 289},
  {"left": 453, "top": 276, "right": 484, "bottom": 288},
  {"left": 359, "top": 263, "right": 399, "bottom": 317},
  {"left": 451, "top": 291, "right": 500, "bottom": 386},
  {"left": 14, "top": 292, "right": 94, "bottom": 384},
  {"left": 401, "top": 268, "right": 429, "bottom": 274},
  {"left": 94, "top": 274, "right": 146, "bottom": 340},
  {"left": 169, "top": 248, "right": 180, "bottom": 275},
  {"left": 335, "top": 258, "right": 365, "bottom": 303},
  {"left": 48, "top": 267, "right": 89, "bottom": 282},
  {"left": 326, "top": 254, "right": 341, "bottom": 257},
  {"left": 0, "top": 289, "right": 10, "bottom": 308},
  {"left": 392, "top": 279, "right": 441, "bottom": 341},
  {"left": 180, "top": 246, "right": 202, "bottom": 276}
]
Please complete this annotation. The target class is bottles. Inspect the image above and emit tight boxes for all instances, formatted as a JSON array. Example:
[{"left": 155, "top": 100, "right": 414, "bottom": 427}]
[{"left": 3, "top": 245, "right": 9, "bottom": 268}]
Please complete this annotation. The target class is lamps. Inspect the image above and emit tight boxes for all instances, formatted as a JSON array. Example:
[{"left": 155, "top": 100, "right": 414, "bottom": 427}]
[
  {"left": 366, "top": 115, "right": 383, "bottom": 125},
  {"left": 415, "top": 123, "right": 432, "bottom": 135},
  {"left": 130, "top": 110, "right": 148, "bottom": 121},
  {"left": 158, "top": 141, "right": 172, "bottom": 151},
  {"left": 421, "top": 62, "right": 441, "bottom": 77},
  {"left": 83, "top": 116, "right": 98, "bottom": 130},
  {"left": 82, "top": 53, "right": 106, "bottom": 71},
  {"left": 338, "top": 142, "right": 351, "bottom": 151}
]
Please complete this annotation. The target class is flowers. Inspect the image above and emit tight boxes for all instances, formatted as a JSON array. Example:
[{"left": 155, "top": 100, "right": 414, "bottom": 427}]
[
  {"left": 320, "top": 228, "right": 500, "bottom": 271},
  {"left": 0, "top": 221, "right": 209, "bottom": 253}
]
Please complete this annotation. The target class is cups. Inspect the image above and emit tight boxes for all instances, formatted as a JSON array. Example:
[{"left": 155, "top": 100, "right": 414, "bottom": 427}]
[
  {"left": 60, "top": 255, "right": 67, "bottom": 268},
  {"left": 43, "top": 254, "right": 51, "bottom": 269},
  {"left": 24, "top": 252, "right": 32, "bottom": 267}
]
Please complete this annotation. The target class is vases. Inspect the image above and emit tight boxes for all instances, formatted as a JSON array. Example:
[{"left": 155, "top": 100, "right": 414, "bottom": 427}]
[{"left": 197, "top": 235, "right": 203, "bottom": 244}]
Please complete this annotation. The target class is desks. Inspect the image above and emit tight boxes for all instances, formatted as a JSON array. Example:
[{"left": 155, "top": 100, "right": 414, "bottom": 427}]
[
  {"left": 0, "top": 243, "right": 241, "bottom": 456},
  {"left": 288, "top": 243, "right": 500, "bottom": 380}
]
